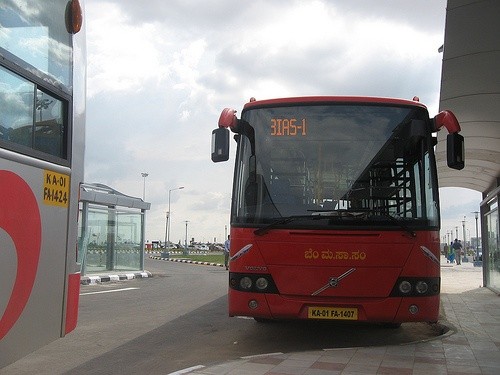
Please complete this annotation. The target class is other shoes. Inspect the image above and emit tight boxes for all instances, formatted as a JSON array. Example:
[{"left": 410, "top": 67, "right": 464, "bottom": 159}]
[
  {"left": 457, "top": 264, "right": 461, "bottom": 265},
  {"left": 226, "top": 267, "right": 228, "bottom": 270}
]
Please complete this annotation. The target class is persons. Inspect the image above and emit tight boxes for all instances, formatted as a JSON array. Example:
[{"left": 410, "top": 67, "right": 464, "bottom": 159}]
[
  {"left": 443, "top": 239, "right": 462, "bottom": 265},
  {"left": 225, "top": 235, "right": 231, "bottom": 270}
]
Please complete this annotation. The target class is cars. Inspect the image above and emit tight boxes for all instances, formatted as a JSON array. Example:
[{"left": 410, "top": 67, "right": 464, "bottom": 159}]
[
  {"left": 473, "top": 245, "right": 491, "bottom": 261},
  {"left": 163, "top": 243, "right": 224, "bottom": 251}
]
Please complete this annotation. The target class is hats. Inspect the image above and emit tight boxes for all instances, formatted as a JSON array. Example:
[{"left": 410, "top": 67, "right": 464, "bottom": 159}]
[{"left": 454, "top": 239, "right": 458, "bottom": 241}]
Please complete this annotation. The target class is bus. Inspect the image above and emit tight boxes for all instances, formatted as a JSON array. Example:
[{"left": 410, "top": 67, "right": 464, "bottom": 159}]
[
  {"left": 211, "top": 94, "right": 466, "bottom": 328},
  {"left": 0, "top": 0, "right": 87, "bottom": 372}
]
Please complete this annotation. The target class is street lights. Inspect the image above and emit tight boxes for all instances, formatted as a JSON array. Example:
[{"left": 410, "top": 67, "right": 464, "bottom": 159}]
[
  {"left": 183, "top": 220, "right": 189, "bottom": 254},
  {"left": 443, "top": 225, "right": 458, "bottom": 251},
  {"left": 140, "top": 172, "right": 149, "bottom": 202},
  {"left": 460, "top": 220, "right": 468, "bottom": 262},
  {"left": 161, "top": 211, "right": 171, "bottom": 258},
  {"left": 471, "top": 211, "right": 480, "bottom": 267},
  {"left": 167, "top": 187, "right": 185, "bottom": 252}
]
